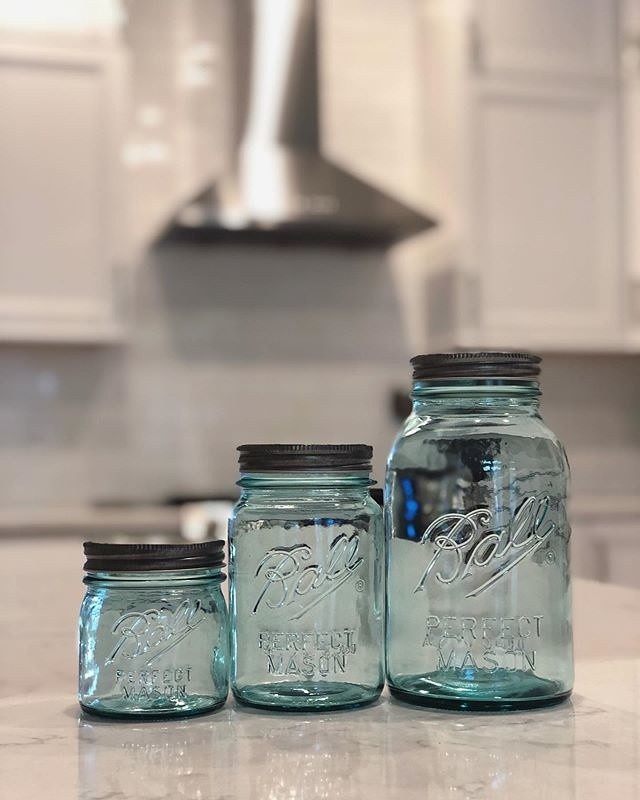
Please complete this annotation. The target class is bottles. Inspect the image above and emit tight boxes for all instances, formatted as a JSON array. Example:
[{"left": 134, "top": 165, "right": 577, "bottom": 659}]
[
  {"left": 382, "top": 350, "right": 576, "bottom": 711},
  {"left": 228, "top": 445, "right": 388, "bottom": 712},
  {"left": 77, "top": 537, "right": 235, "bottom": 716}
]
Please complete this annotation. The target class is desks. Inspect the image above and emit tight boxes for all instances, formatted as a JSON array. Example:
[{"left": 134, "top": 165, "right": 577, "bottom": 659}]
[{"left": 0, "top": 523, "right": 640, "bottom": 800}]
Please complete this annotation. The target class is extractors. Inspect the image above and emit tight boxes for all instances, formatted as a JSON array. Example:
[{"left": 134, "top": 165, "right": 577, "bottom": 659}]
[{"left": 156, "top": 1, "right": 436, "bottom": 248}]
